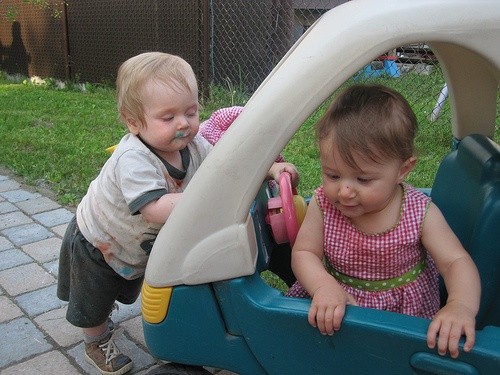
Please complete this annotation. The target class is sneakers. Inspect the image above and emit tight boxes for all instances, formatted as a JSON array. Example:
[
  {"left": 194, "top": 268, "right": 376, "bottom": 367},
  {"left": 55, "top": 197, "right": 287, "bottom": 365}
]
[{"left": 84, "top": 334, "right": 133, "bottom": 375}]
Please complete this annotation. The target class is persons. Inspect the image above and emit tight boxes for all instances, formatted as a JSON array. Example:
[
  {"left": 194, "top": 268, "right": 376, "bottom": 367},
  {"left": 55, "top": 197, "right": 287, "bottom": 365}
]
[
  {"left": 283, "top": 84, "right": 482, "bottom": 360},
  {"left": 56, "top": 51, "right": 299, "bottom": 375}
]
